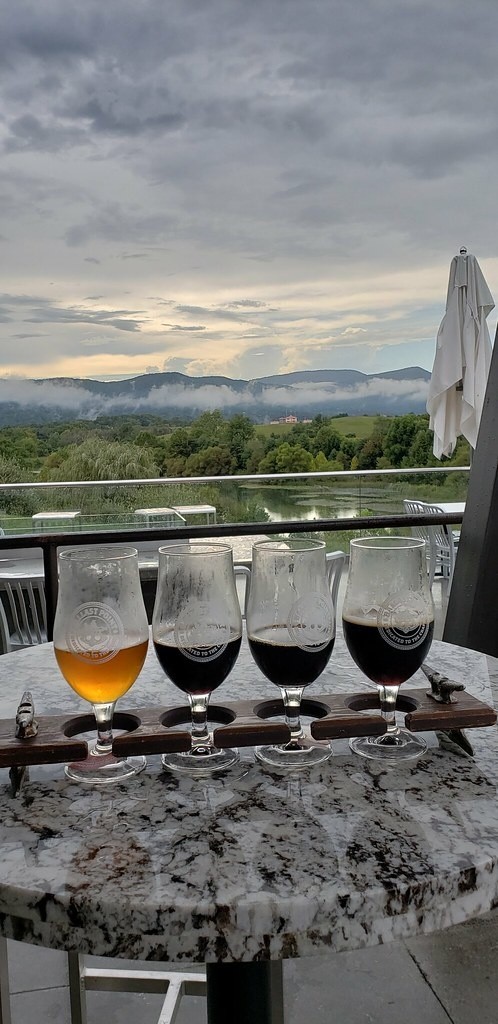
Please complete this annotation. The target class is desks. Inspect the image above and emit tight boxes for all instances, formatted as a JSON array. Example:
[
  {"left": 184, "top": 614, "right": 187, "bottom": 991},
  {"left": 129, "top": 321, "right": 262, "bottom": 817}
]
[
  {"left": 404, "top": 502, "right": 467, "bottom": 576},
  {"left": 31, "top": 512, "right": 82, "bottom": 532},
  {"left": 172, "top": 504, "right": 216, "bottom": 525},
  {"left": 0, "top": 620, "right": 498, "bottom": 1023},
  {"left": 58, "top": 534, "right": 290, "bottom": 627},
  {"left": 134, "top": 507, "right": 175, "bottom": 528}
]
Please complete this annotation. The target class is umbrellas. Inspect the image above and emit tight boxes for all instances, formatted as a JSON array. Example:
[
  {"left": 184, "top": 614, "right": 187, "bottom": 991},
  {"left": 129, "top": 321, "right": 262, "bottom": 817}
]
[{"left": 426, "top": 246, "right": 496, "bottom": 460}]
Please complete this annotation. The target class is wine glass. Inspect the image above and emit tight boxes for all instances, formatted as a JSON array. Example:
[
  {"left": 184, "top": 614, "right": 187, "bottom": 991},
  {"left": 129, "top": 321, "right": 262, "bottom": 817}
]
[
  {"left": 152, "top": 541, "right": 243, "bottom": 773},
  {"left": 244, "top": 538, "right": 336, "bottom": 768},
  {"left": 342, "top": 536, "right": 435, "bottom": 761},
  {"left": 53, "top": 546, "right": 149, "bottom": 784}
]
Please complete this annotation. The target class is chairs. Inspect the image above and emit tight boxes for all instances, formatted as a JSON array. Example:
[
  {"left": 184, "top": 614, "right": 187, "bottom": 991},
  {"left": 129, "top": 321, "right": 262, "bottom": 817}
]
[
  {"left": 326, "top": 550, "right": 347, "bottom": 621},
  {"left": 402, "top": 499, "right": 431, "bottom": 579},
  {"left": 0, "top": 576, "right": 50, "bottom": 653},
  {"left": 422, "top": 505, "right": 459, "bottom": 596},
  {"left": 229, "top": 565, "right": 251, "bottom": 621}
]
